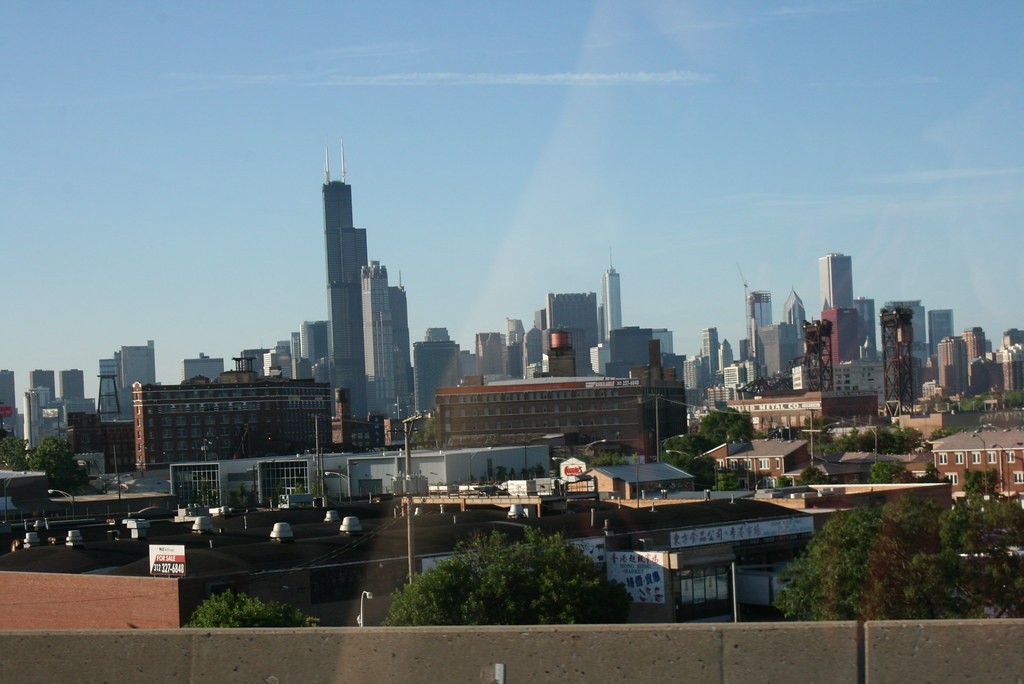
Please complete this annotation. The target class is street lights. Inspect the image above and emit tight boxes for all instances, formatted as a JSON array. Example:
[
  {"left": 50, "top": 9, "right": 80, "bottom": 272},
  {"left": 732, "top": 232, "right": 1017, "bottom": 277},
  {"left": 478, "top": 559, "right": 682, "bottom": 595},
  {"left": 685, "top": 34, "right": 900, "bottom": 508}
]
[
  {"left": 324, "top": 471, "right": 351, "bottom": 503},
  {"left": 990, "top": 441, "right": 1023, "bottom": 504},
  {"left": 581, "top": 439, "right": 607, "bottom": 458},
  {"left": 339, "top": 462, "right": 359, "bottom": 503},
  {"left": 48, "top": 489, "right": 75, "bottom": 520},
  {"left": 970, "top": 433, "right": 987, "bottom": 495},
  {"left": 659, "top": 435, "right": 684, "bottom": 462},
  {"left": 469, "top": 447, "right": 494, "bottom": 486},
  {"left": 361, "top": 590, "right": 372, "bottom": 627}
]
